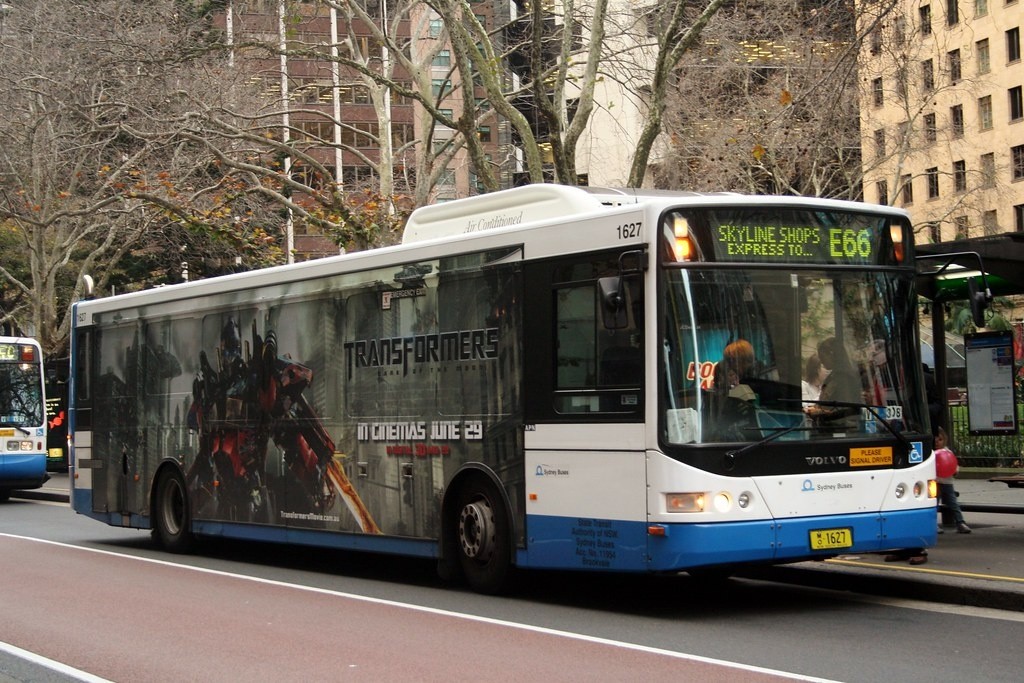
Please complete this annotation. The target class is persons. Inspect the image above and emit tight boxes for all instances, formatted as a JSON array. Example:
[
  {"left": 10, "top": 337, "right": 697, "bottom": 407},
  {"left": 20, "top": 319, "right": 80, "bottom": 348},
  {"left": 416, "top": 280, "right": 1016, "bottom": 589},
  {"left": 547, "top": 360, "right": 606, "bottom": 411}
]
[
  {"left": 799, "top": 351, "right": 841, "bottom": 405},
  {"left": 798, "top": 333, "right": 864, "bottom": 420},
  {"left": 930, "top": 429, "right": 974, "bottom": 534}
]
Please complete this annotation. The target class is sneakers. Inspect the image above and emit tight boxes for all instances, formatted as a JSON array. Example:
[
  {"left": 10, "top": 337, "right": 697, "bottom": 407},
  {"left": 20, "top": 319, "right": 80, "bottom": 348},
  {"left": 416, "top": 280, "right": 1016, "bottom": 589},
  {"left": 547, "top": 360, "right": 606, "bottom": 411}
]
[
  {"left": 937, "top": 526, "right": 944, "bottom": 533},
  {"left": 957, "top": 523, "right": 971, "bottom": 533}
]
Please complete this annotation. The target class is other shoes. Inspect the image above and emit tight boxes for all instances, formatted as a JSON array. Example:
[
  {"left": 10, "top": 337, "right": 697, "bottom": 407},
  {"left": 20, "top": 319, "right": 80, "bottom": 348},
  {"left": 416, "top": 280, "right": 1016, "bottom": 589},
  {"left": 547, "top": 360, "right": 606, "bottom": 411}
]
[
  {"left": 909, "top": 554, "right": 927, "bottom": 564},
  {"left": 885, "top": 552, "right": 908, "bottom": 561}
]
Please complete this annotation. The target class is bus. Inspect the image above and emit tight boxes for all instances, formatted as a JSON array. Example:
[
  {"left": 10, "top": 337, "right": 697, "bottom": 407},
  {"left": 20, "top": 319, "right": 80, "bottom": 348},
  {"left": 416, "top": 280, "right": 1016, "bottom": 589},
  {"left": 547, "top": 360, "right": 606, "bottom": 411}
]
[
  {"left": 0, "top": 336, "right": 49, "bottom": 505},
  {"left": 71, "top": 184, "right": 997, "bottom": 599}
]
